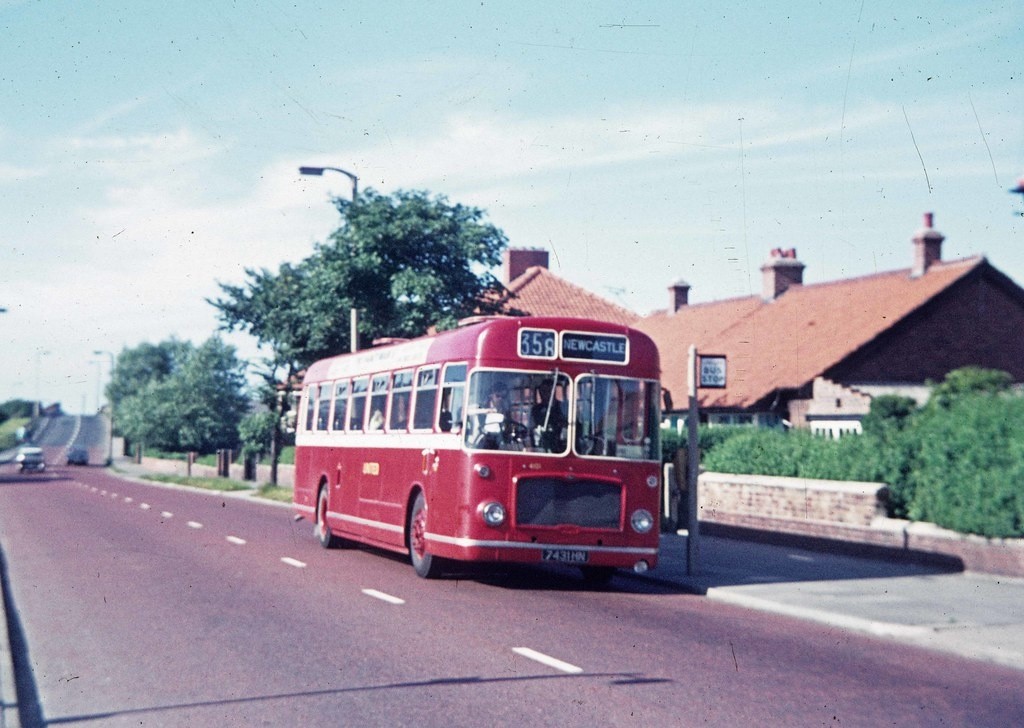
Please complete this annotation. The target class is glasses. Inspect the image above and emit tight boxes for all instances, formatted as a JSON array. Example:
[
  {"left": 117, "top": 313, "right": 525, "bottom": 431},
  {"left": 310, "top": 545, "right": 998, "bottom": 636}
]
[{"left": 495, "top": 393, "right": 506, "bottom": 399}]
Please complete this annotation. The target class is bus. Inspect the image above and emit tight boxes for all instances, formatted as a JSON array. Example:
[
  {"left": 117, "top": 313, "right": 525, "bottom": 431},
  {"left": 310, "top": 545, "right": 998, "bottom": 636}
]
[{"left": 292, "top": 314, "right": 671, "bottom": 582}]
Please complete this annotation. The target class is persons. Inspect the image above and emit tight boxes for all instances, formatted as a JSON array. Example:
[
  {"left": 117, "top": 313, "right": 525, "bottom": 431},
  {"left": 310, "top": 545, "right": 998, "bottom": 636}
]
[
  {"left": 531, "top": 378, "right": 566, "bottom": 454},
  {"left": 467, "top": 382, "right": 509, "bottom": 451},
  {"left": 306, "top": 387, "right": 463, "bottom": 434}
]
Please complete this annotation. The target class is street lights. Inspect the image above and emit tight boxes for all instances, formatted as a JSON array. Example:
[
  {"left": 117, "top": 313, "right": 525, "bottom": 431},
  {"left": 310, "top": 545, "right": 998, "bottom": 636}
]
[
  {"left": 298, "top": 164, "right": 359, "bottom": 354},
  {"left": 95, "top": 350, "right": 116, "bottom": 379}
]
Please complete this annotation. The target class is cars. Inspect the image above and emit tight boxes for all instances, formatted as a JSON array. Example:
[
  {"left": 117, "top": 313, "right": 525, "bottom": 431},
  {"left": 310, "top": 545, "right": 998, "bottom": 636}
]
[
  {"left": 65, "top": 446, "right": 89, "bottom": 466},
  {"left": 15, "top": 446, "right": 45, "bottom": 472}
]
